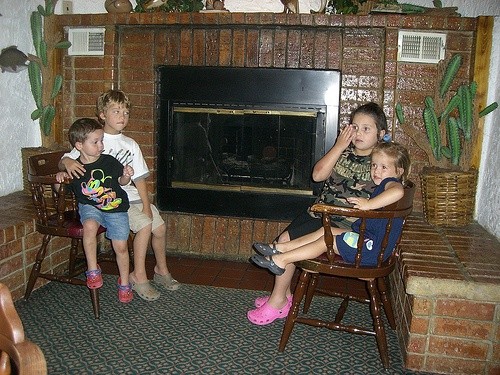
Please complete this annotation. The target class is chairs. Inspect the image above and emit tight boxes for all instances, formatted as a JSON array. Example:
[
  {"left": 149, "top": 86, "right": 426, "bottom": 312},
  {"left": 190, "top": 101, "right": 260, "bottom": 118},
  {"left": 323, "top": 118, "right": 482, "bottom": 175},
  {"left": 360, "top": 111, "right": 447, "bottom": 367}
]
[
  {"left": 24, "top": 151, "right": 134, "bottom": 319},
  {"left": 280, "top": 180, "right": 416, "bottom": 372}
]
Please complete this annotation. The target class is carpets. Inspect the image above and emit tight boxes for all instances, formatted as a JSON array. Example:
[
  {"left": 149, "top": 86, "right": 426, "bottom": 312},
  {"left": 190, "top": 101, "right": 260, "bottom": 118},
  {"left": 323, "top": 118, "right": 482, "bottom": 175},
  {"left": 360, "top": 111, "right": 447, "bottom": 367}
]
[{"left": 14, "top": 273, "right": 444, "bottom": 375}]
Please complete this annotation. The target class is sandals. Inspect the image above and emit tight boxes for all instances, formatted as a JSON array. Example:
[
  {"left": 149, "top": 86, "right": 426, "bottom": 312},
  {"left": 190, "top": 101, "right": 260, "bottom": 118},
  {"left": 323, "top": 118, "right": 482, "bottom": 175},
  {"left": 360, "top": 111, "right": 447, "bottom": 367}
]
[
  {"left": 247, "top": 302, "right": 292, "bottom": 325},
  {"left": 254, "top": 293, "right": 293, "bottom": 308}
]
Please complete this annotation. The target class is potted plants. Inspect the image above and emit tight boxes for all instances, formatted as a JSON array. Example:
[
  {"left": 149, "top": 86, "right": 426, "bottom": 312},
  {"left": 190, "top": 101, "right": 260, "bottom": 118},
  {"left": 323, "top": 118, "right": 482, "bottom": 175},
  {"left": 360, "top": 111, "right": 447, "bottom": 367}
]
[
  {"left": 398, "top": 54, "right": 499, "bottom": 228},
  {"left": 21, "top": 0, "right": 72, "bottom": 197}
]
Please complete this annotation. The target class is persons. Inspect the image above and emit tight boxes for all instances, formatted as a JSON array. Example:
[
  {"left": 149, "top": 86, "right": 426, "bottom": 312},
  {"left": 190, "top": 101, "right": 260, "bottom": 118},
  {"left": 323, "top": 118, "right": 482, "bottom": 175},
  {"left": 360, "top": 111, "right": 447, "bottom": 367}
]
[
  {"left": 56, "top": 118, "right": 134, "bottom": 302},
  {"left": 58, "top": 89, "right": 180, "bottom": 301},
  {"left": 251, "top": 134, "right": 411, "bottom": 276},
  {"left": 247, "top": 103, "right": 388, "bottom": 325}
]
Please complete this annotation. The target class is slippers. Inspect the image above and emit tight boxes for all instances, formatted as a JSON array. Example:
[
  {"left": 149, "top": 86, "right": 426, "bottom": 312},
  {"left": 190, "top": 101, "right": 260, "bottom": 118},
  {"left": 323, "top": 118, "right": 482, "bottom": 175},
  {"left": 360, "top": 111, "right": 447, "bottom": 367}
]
[
  {"left": 86, "top": 264, "right": 103, "bottom": 289},
  {"left": 117, "top": 278, "right": 132, "bottom": 304},
  {"left": 251, "top": 254, "right": 286, "bottom": 276},
  {"left": 154, "top": 272, "right": 180, "bottom": 291},
  {"left": 253, "top": 242, "right": 282, "bottom": 256},
  {"left": 129, "top": 274, "right": 161, "bottom": 301}
]
